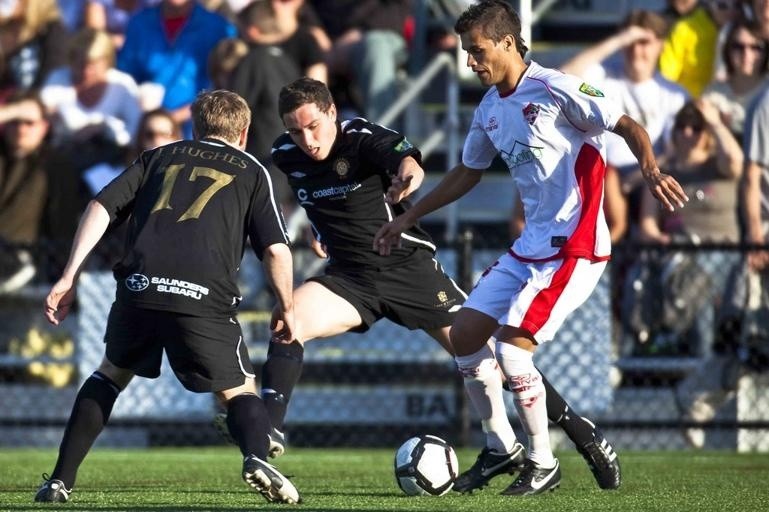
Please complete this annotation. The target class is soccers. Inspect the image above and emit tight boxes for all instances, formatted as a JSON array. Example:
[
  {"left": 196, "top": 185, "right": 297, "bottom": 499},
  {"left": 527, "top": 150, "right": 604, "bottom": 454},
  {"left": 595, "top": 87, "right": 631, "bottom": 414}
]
[{"left": 394, "top": 434, "right": 459, "bottom": 497}]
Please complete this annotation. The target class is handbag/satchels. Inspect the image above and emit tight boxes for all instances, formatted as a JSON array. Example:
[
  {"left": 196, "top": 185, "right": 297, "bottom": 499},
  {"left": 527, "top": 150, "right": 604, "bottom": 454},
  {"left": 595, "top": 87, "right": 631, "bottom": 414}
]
[
  {"left": 613, "top": 246, "right": 714, "bottom": 349},
  {"left": 714, "top": 263, "right": 769, "bottom": 372}
]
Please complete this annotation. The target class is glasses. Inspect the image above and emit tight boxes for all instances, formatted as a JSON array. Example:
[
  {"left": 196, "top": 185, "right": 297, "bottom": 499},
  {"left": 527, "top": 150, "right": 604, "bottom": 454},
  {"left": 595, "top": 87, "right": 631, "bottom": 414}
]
[
  {"left": 675, "top": 109, "right": 704, "bottom": 132},
  {"left": 725, "top": 39, "right": 765, "bottom": 51}
]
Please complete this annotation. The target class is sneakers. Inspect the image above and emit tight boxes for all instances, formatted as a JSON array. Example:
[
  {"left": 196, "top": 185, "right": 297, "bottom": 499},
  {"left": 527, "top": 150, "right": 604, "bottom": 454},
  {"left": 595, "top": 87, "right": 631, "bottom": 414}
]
[
  {"left": 500, "top": 458, "right": 562, "bottom": 496},
  {"left": 242, "top": 454, "right": 300, "bottom": 505},
  {"left": 576, "top": 417, "right": 621, "bottom": 489},
  {"left": 676, "top": 379, "right": 705, "bottom": 448},
  {"left": 212, "top": 413, "right": 287, "bottom": 458},
  {"left": 452, "top": 440, "right": 528, "bottom": 495},
  {"left": 34, "top": 473, "right": 73, "bottom": 504}
]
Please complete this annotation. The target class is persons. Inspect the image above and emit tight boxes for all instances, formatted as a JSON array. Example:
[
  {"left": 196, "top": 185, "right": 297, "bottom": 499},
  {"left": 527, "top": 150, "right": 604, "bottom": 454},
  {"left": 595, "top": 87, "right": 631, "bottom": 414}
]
[
  {"left": 0, "top": 0, "right": 769, "bottom": 447},
  {"left": 373, "top": 3, "right": 690, "bottom": 496},
  {"left": 34, "top": 89, "right": 304, "bottom": 505},
  {"left": 212, "top": 77, "right": 622, "bottom": 489}
]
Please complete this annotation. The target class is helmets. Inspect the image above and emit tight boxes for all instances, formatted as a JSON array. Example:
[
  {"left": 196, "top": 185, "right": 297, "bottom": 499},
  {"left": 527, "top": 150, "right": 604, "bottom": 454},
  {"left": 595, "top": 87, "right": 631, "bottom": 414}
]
[{"left": 67, "top": 28, "right": 115, "bottom": 59}]
